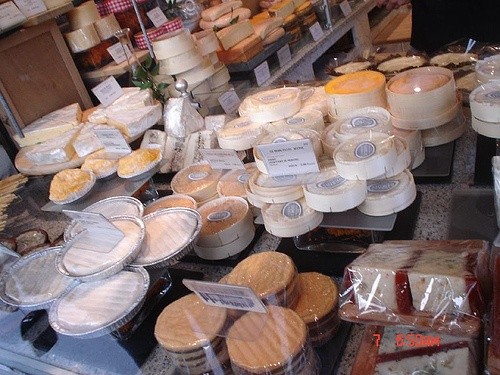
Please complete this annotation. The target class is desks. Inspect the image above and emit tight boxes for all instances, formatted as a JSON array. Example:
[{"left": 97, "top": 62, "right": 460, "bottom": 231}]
[{"left": 0, "top": 105, "right": 500, "bottom": 375}]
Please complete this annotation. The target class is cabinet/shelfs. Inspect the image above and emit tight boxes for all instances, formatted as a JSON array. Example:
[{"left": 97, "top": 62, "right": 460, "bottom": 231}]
[
  {"left": 0, "top": 19, "right": 96, "bottom": 140},
  {"left": 261, "top": 0, "right": 401, "bottom": 89}
]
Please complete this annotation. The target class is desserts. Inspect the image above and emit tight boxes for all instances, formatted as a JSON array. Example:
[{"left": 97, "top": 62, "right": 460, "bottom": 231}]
[{"left": 1, "top": 0, "right": 499, "bottom": 375}]
